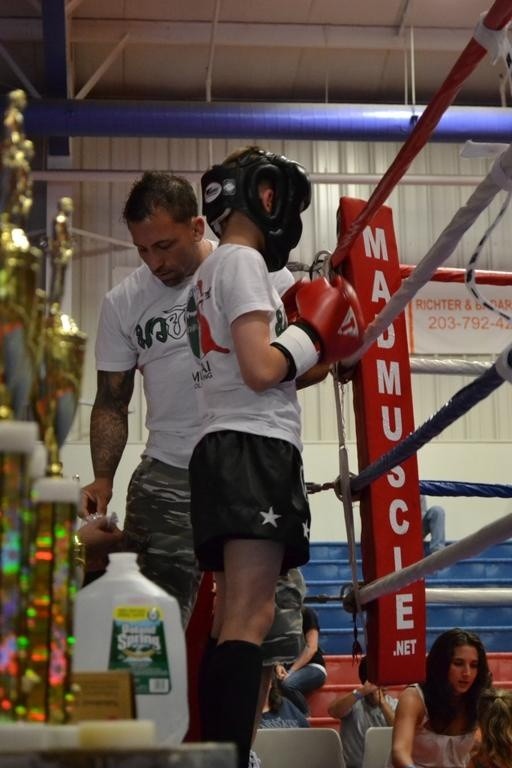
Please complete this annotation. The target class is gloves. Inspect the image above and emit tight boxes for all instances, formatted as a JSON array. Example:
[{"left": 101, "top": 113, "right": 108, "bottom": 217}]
[{"left": 281, "top": 277, "right": 366, "bottom": 367}]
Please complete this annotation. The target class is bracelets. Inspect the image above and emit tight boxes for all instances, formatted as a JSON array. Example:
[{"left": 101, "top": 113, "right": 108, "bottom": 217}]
[
  {"left": 287, "top": 668, "right": 296, "bottom": 676},
  {"left": 353, "top": 688, "right": 365, "bottom": 699}
]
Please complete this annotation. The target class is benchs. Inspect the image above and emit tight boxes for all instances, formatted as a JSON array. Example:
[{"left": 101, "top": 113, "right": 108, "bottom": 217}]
[{"left": 294, "top": 541, "right": 512, "bottom": 727}]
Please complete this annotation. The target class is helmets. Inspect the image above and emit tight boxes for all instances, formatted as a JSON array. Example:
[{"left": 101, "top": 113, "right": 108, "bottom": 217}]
[{"left": 200, "top": 153, "right": 312, "bottom": 272}]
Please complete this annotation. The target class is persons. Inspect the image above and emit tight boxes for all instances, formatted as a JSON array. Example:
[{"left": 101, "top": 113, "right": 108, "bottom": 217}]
[
  {"left": 329, "top": 654, "right": 400, "bottom": 768},
  {"left": 73, "top": 514, "right": 124, "bottom": 588},
  {"left": 464, "top": 684, "right": 512, "bottom": 768},
  {"left": 266, "top": 604, "right": 328, "bottom": 726},
  {"left": 184, "top": 144, "right": 368, "bottom": 767},
  {"left": 387, "top": 628, "right": 495, "bottom": 768},
  {"left": 76, "top": 171, "right": 314, "bottom": 767}
]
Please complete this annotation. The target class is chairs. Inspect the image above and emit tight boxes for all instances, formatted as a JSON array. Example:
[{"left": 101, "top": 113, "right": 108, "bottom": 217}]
[
  {"left": 254, "top": 727, "right": 346, "bottom": 768},
  {"left": 363, "top": 728, "right": 395, "bottom": 768}
]
